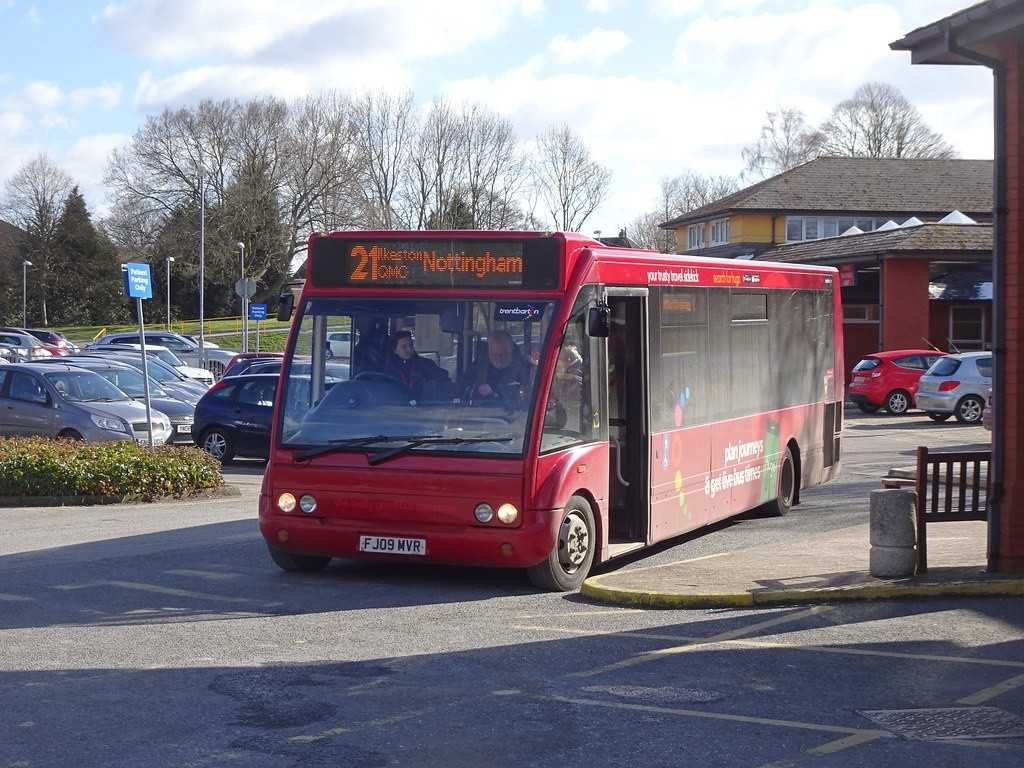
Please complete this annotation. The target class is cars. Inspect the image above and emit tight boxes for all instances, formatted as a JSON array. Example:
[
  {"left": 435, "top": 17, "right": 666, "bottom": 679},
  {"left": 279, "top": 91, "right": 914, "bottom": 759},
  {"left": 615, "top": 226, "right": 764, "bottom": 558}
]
[
  {"left": 222, "top": 353, "right": 313, "bottom": 378},
  {"left": 983, "top": 387, "right": 993, "bottom": 431},
  {"left": 97, "top": 332, "right": 246, "bottom": 382},
  {"left": 56, "top": 362, "right": 196, "bottom": 441},
  {"left": 191, "top": 374, "right": 345, "bottom": 466},
  {"left": 23, "top": 357, "right": 201, "bottom": 408},
  {"left": 915, "top": 351, "right": 994, "bottom": 426},
  {"left": 238, "top": 362, "right": 350, "bottom": 391},
  {"left": 0, "top": 363, "right": 175, "bottom": 446},
  {"left": 162, "top": 335, "right": 219, "bottom": 349},
  {"left": 849, "top": 350, "right": 950, "bottom": 417},
  {"left": 325, "top": 332, "right": 360, "bottom": 361},
  {"left": 0, "top": 327, "right": 80, "bottom": 384},
  {"left": 69, "top": 344, "right": 216, "bottom": 396}
]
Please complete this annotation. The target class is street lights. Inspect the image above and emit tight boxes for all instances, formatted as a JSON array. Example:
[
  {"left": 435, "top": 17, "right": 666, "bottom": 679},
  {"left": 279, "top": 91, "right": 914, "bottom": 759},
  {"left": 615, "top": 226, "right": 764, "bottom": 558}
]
[
  {"left": 237, "top": 242, "right": 245, "bottom": 352},
  {"left": 197, "top": 164, "right": 207, "bottom": 369},
  {"left": 166, "top": 257, "right": 174, "bottom": 332},
  {"left": 593, "top": 230, "right": 601, "bottom": 242},
  {"left": 23, "top": 261, "right": 33, "bottom": 329}
]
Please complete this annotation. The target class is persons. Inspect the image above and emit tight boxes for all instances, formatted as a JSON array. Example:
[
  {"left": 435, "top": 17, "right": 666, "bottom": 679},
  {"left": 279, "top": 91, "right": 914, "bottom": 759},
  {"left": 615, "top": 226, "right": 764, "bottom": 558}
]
[
  {"left": 467, "top": 329, "right": 538, "bottom": 410},
  {"left": 384, "top": 330, "right": 450, "bottom": 402},
  {"left": 555, "top": 345, "right": 583, "bottom": 410}
]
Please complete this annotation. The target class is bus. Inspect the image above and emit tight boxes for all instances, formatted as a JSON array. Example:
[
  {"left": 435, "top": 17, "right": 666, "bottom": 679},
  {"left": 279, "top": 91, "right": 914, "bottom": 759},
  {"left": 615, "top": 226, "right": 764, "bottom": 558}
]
[{"left": 259, "top": 231, "right": 845, "bottom": 592}]
